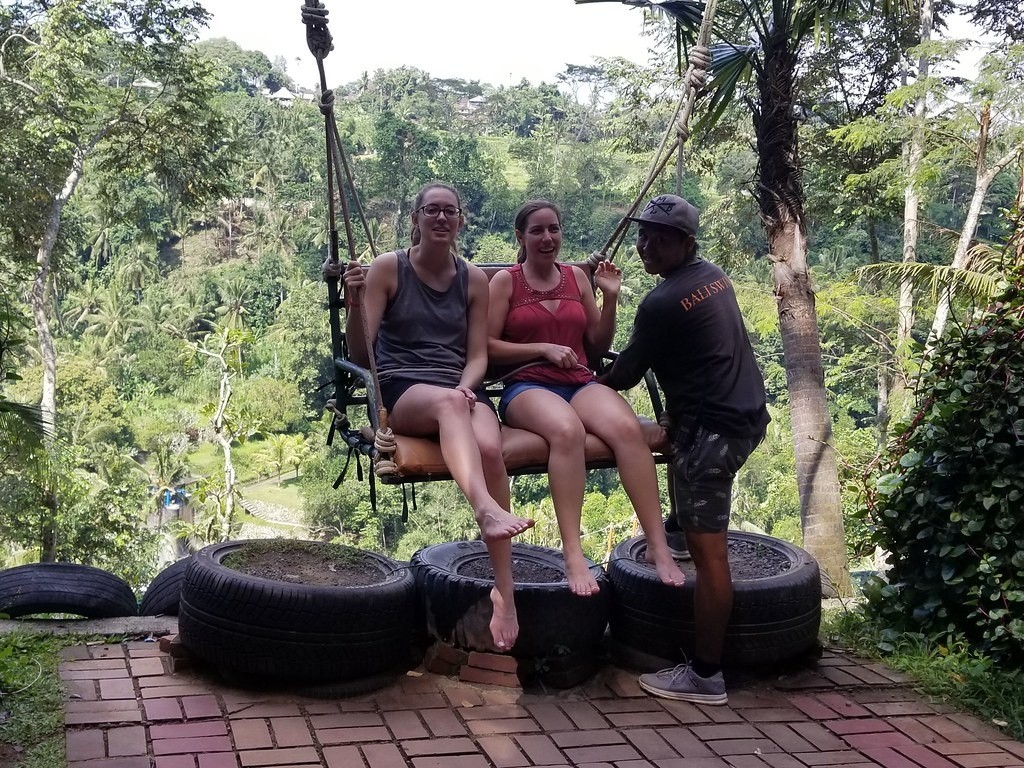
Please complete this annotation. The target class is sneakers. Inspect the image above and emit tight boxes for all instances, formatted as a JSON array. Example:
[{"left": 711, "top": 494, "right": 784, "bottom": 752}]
[
  {"left": 661, "top": 517, "right": 691, "bottom": 559},
  {"left": 638, "top": 648, "right": 728, "bottom": 705}
]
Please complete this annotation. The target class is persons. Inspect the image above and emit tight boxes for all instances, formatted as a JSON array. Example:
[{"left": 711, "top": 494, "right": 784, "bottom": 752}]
[
  {"left": 595, "top": 193, "right": 772, "bottom": 706},
  {"left": 486, "top": 201, "right": 687, "bottom": 597},
  {"left": 343, "top": 183, "right": 536, "bottom": 650}
]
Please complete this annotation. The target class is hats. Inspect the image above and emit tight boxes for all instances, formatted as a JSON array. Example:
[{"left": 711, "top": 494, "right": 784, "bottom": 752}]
[{"left": 625, "top": 195, "right": 699, "bottom": 235}]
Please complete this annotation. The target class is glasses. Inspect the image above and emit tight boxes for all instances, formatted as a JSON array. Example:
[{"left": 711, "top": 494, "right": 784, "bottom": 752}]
[{"left": 416, "top": 203, "right": 462, "bottom": 219}]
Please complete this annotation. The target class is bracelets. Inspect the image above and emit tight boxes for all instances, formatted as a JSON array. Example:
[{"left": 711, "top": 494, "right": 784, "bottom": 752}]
[{"left": 348, "top": 300, "right": 360, "bottom": 308}]
